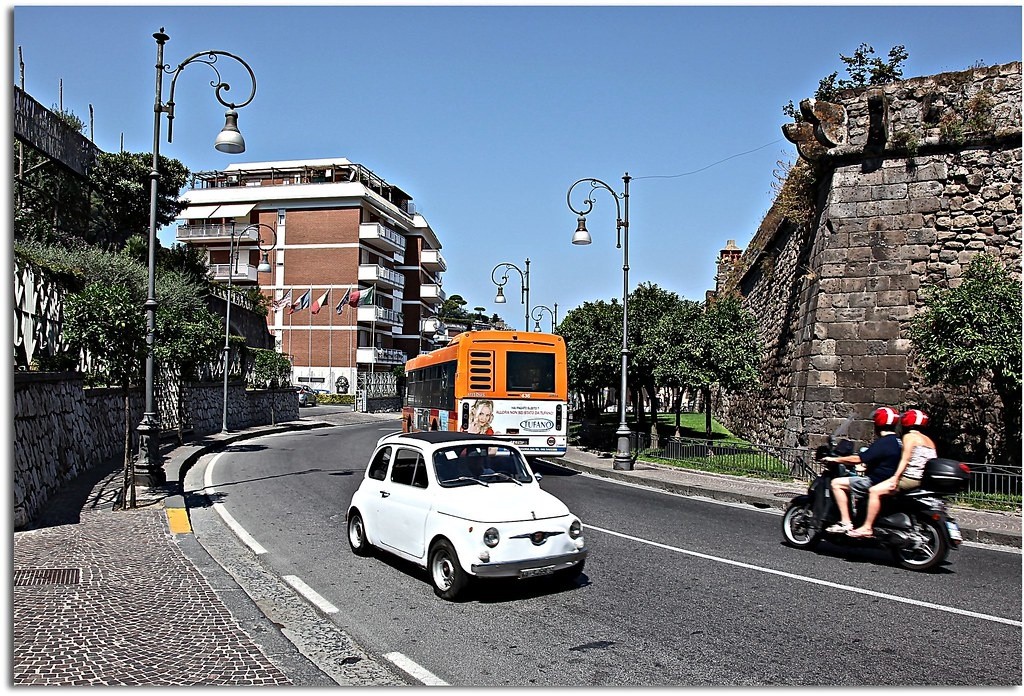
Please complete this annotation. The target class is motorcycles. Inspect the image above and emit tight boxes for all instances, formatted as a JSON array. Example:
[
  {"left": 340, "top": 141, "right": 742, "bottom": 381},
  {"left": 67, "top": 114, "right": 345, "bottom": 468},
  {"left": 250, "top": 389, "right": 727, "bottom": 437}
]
[{"left": 782, "top": 411, "right": 974, "bottom": 572}]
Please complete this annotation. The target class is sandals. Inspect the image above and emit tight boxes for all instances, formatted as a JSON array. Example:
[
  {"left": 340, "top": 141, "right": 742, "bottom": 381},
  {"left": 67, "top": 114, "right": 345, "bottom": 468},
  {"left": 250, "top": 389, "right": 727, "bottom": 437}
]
[
  {"left": 846, "top": 527, "right": 874, "bottom": 538},
  {"left": 825, "top": 521, "right": 853, "bottom": 534}
]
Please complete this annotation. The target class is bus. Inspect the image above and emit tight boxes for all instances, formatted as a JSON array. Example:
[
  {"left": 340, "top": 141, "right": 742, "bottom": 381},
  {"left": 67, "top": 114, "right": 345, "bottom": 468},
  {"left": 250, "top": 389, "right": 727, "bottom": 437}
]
[{"left": 398, "top": 330, "right": 568, "bottom": 465}]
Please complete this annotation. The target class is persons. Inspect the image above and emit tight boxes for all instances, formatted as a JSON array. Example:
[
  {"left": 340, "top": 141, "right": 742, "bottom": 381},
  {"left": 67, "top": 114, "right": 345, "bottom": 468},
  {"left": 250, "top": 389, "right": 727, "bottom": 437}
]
[
  {"left": 468, "top": 457, "right": 495, "bottom": 481},
  {"left": 845, "top": 408, "right": 938, "bottom": 538},
  {"left": 818, "top": 406, "right": 903, "bottom": 533},
  {"left": 460, "top": 399, "right": 494, "bottom": 435}
]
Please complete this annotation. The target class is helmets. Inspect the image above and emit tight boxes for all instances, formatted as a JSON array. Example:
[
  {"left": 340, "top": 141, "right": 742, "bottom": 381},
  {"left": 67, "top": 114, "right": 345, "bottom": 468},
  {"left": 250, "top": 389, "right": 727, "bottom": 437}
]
[
  {"left": 873, "top": 407, "right": 899, "bottom": 428},
  {"left": 901, "top": 409, "right": 928, "bottom": 428}
]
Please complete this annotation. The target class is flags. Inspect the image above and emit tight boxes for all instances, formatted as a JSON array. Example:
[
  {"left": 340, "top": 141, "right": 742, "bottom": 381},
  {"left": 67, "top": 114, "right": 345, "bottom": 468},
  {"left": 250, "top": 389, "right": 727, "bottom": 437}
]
[
  {"left": 335, "top": 287, "right": 350, "bottom": 316},
  {"left": 286, "top": 288, "right": 310, "bottom": 315},
  {"left": 349, "top": 286, "right": 374, "bottom": 308},
  {"left": 309, "top": 288, "right": 331, "bottom": 314},
  {"left": 271, "top": 288, "right": 291, "bottom": 313}
]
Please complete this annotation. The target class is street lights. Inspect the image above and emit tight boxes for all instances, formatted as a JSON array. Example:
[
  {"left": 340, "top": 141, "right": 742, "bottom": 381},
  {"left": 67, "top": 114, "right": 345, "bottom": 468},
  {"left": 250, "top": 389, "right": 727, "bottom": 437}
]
[
  {"left": 531, "top": 305, "right": 556, "bottom": 335},
  {"left": 130, "top": 26, "right": 257, "bottom": 494},
  {"left": 569, "top": 171, "right": 633, "bottom": 471},
  {"left": 491, "top": 259, "right": 531, "bottom": 332},
  {"left": 214, "top": 219, "right": 278, "bottom": 438}
]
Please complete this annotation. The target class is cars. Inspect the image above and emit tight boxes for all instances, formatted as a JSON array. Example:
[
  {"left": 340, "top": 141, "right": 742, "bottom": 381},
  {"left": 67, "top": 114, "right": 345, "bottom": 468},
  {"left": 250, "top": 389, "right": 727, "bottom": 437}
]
[
  {"left": 343, "top": 430, "right": 591, "bottom": 599},
  {"left": 290, "top": 384, "right": 317, "bottom": 407}
]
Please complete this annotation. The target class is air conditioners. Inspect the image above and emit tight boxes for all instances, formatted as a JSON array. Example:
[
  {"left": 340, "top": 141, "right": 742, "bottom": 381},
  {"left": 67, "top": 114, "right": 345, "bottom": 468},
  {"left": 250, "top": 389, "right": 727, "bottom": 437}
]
[{"left": 227, "top": 175, "right": 238, "bottom": 184}]
